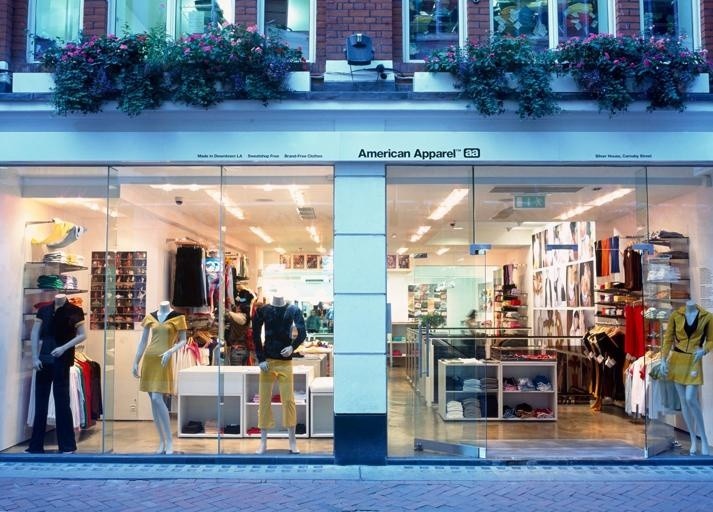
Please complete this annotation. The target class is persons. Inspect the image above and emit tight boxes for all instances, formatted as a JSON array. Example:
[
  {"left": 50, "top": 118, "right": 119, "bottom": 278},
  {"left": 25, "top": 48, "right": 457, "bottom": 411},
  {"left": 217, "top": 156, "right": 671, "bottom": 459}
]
[
  {"left": 132, "top": 301, "right": 187, "bottom": 455},
  {"left": 661, "top": 300, "right": 713, "bottom": 456},
  {"left": 307, "top": 310, "right": 321, "bottom": 333},
  {"left": 225, "top": 303, "right": 250, "bottom": 365},
  {"left": 531, "top": 221, "right": 591, "bottom": 393},
  {"left": 252, "top": 293, "right": 307, "bottom": 454},
  {"left": 22, "top": 294, "right": 87, "bottom": 455}
]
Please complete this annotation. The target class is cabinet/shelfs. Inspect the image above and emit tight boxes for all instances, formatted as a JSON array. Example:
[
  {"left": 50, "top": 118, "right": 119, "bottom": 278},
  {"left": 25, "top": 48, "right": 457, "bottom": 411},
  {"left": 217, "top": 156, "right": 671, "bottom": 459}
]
[
  {"left": 438, "top": 358, "right": 557, "bottom": 422},
  {"left": 494, "top": 290, "right": 528, "bottom": 335},
  {"left": 176, "top": 330, "right": 334, "bottom": 438},
  {"left": 593, "top": 231, "right": 690, "bottom": 353},
  {"left": 21, "top": 260, "right": 88, "bottom": 356},
  {"left": 386, "top": 322, "right": 420, "bottom": 369}
]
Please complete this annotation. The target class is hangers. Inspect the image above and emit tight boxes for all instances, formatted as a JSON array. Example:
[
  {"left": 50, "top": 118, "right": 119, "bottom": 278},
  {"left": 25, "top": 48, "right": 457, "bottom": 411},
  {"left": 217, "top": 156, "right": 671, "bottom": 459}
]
[
  {"left": 72, "top": 345, "right": 93, "bottom": 365},
  {"left": 644, "top": 344, "right": 662, "bottom": 360}
]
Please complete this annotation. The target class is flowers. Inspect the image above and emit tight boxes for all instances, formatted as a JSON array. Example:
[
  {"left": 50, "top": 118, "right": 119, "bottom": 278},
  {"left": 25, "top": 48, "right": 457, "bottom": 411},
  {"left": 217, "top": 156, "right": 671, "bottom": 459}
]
[
  {"left": 424, "top": 29, "right": 712, "bottom": 122},
  {"left": 26, "top": 7, "right": 307, "bottom": 118}
]
